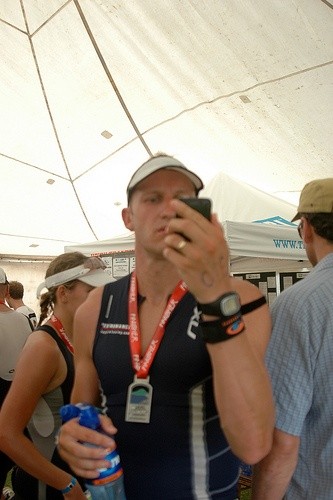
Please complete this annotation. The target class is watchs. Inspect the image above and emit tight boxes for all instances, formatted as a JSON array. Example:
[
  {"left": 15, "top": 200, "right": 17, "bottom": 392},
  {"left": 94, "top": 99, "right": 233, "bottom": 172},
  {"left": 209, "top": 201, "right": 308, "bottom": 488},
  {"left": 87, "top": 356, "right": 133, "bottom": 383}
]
[{"left": 196, "top": 291, "right": 242, "bottom": 317}]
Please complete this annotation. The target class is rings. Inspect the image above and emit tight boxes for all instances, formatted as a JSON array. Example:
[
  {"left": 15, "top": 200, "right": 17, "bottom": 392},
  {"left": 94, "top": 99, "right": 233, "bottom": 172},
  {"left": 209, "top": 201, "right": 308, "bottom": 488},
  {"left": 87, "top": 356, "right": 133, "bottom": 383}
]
[{"left": 178, "top": 241, "right": 186, "bottom": 250}]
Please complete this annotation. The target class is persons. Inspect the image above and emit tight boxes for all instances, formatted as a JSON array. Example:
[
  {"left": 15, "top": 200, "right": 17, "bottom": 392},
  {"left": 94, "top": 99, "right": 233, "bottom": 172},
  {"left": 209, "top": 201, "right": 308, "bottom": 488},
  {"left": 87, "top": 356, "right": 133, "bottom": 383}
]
[
  {"left": 0, "top": 267, "right": 40, "bottom": 500},
  {"left": 248, "top": 177, "right": 333, "bottom": 500},
  {"left": 0, "top": 252, "right": 117, "bottom": 500},
  {"left": 56, "top": 155, "right": 274, "bottom": 500}
]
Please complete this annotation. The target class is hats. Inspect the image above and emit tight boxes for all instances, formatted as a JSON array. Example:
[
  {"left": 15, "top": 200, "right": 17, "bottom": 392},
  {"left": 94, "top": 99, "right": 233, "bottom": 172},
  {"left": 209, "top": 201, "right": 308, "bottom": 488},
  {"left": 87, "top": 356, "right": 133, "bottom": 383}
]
[
  {"left": 291, "top": 178, "right": 333, "bottom": 222},
  {"left": 37, "top": 256, "right": 117, "bottom": 301},
  {"left": 126, "top": 156, "right": 203, "bottom": 207},
  {"left": 0, "top": 267, "right": 10, "bottom": 284}
]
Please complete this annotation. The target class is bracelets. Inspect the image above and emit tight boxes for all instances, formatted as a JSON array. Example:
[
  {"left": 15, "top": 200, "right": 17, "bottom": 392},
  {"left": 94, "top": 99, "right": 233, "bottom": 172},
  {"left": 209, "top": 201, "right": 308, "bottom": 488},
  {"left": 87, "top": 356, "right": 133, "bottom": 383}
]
[
  {"left": 199, "top": 312, "right": 246, "bottom": 343},
  {"left": 60, "top": 477, "right": 76, "bottom": 493}
]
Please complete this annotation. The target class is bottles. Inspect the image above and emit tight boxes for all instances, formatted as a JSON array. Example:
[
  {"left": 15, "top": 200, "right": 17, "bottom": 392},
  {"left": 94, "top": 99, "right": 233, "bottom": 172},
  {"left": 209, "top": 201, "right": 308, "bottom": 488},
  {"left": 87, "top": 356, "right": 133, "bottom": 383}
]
[
  {"left": 78, "top": 405, "right": 127, "bottom": 500},
  {"left": 59, "top": 402, "right": 104, "bottom": 423}
]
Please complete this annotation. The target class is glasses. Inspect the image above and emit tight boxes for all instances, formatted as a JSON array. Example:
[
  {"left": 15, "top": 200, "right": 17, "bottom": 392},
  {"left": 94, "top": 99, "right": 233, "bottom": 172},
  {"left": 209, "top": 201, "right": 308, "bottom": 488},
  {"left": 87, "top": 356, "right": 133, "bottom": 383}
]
[
  {"left": 297, "top": 221, "right": 305, "bottom": 239},
  {"left": 52, "top": 257, "right": 107, "bottom": 285}
]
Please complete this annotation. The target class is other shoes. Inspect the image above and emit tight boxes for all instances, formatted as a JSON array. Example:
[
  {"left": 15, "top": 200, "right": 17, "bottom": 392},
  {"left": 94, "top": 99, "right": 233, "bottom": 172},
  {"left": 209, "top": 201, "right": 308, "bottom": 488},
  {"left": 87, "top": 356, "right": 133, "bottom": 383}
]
[{"left": 2, "top": 487, "right": 15, "bottom": 500}]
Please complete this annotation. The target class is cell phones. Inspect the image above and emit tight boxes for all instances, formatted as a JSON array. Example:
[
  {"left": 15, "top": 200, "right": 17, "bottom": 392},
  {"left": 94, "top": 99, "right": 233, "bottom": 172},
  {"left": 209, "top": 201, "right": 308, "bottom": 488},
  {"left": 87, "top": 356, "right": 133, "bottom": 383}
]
[{"left": 173, "top": 198, "right": 211, "bottom": 242}]
